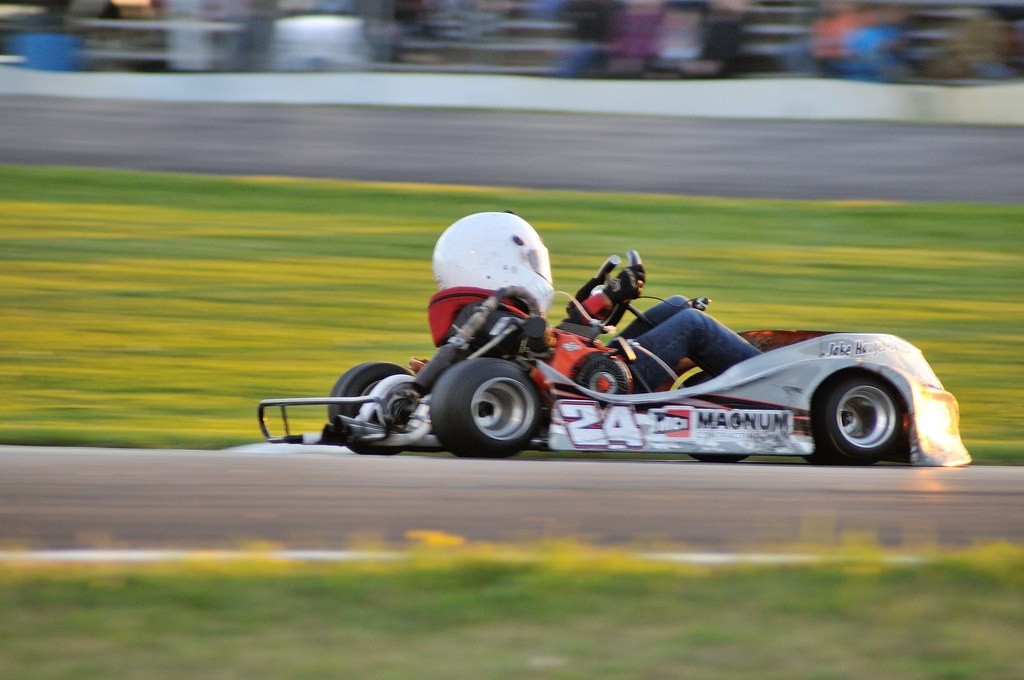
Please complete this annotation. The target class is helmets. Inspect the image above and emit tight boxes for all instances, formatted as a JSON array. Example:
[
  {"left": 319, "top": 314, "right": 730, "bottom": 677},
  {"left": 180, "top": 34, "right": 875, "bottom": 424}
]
[{"left": 431, "top": 211, "right": 554, "bottom": 319}]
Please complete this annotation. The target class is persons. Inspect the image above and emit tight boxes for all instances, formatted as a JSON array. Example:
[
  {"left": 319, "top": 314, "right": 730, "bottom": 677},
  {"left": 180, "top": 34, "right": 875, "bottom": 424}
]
[{"left": 428, "top": 211, "right": 765, "bottom": 395}]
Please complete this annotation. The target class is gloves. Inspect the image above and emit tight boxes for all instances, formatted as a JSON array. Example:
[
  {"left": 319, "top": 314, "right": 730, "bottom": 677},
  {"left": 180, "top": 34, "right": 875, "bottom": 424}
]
[{"left": 603, "top": 264, "right": 645, "bottom": 304}]
